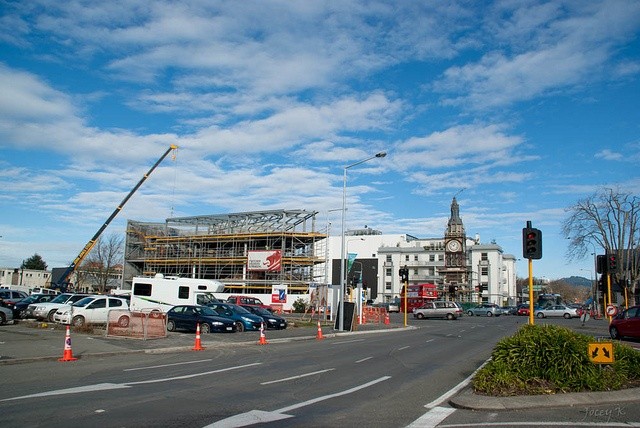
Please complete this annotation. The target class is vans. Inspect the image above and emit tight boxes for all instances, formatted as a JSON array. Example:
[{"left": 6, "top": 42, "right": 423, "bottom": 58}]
[{"left": 0, "top": 290, "right": 27, "bottom": 305}]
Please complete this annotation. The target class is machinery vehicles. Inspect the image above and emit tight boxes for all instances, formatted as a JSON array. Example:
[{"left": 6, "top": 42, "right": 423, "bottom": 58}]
[{"left": 130, "top": 273, "right": 225, "bottom": 318}]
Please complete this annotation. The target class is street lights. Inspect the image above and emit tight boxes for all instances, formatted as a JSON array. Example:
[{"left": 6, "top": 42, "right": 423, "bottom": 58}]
[{"left": 339, "top": 153, "right": 386, "bottom": 331}]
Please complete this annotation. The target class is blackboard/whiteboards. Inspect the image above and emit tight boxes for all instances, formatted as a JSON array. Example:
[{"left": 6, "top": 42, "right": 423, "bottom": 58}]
[{"left": 334, "top": 301, "right": 356, "bottom": 332}]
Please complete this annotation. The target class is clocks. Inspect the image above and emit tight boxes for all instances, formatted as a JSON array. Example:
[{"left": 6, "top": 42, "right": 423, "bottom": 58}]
[{"left": 448, "top": 239, "right": 460, "bottom": 252}]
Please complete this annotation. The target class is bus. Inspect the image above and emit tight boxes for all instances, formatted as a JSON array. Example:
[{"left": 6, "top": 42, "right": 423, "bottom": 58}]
[{"left": 400, "top": 284, "right": 437, "bottom": 312}]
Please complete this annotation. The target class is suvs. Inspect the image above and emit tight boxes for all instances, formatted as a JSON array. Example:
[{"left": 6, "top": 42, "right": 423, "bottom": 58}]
[{"left": 226, "top": 296, "right": 272, "bottom": 313}]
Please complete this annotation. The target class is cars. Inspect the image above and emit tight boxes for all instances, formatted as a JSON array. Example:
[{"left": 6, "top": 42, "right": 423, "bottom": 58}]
[
  {"left": 242, "top": 305, "right": 287, "bottom": 330},
  {"left": 413, "top": 301, "right": 459, "bottom": 320},
  {"left": 466, "top": 303, "right": 502, "bottom": 317},
  {"left": 518, "top": 305, "right": 530, "bottom": 315},
  {"left": 25, "top": 293, "right": 91, "bottom": 322},
  {"left": 501, "top": 306, "right": 516, "bottom": 316},
  {"left": 165, "top": 306, "right": 236, "bottom": 333},
  {"left": 206, "top": 302, "right": 267, "bottom": 330},
  {"left": 535, "top": 305, "right": 580, "bottom": 319},
  {"left": 0, "top": 306, "right": 13, "bottom": 325},
  {"left": 609, "top": 306, "right": 640, "bottom": 339},
  {"left": 52, "top": 295, "right": 130, "bottom": 327},
  {"left": 455, "top": 302, "right": 464, "bottom": 317},
  {"left": 1, "top": 298, "right": 16, "bottom": 306},
  {"left": 388, "top": 303, "right": 398, "bottom": 313},
  {"left": 13, "top": 294, "right": 55, "bottom": 319}
]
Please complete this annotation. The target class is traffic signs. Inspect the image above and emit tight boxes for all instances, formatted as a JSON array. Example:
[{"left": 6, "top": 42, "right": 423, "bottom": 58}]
[{"left": 587, "top": 338, "right": 614, "bottom": 375}]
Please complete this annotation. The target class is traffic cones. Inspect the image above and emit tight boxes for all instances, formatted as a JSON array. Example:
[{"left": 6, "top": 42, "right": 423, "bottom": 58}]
[
  {"left": 192, "top": 323, "right": 205, "bottom": 350},
  {"left": 316, "top": 320, "right": 327, "bottom": 339},
  {"left": 57, "top": 326, "right": 77, "bottom": 361},
  {"left": 256, "top": 323, "right": 271, "bottom": 344}
]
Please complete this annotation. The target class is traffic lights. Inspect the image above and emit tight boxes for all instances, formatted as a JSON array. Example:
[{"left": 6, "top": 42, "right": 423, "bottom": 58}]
[
  {"left": 523, "top": 228, "right": 542, "bottom": 259},
  {"left": 606, "top": 253, "right": 617, "bottom": 274},
  {"left": 399, "top": 268, "right": 408, "bottom": 283},
  {"left": 597, "top": 255, "right": 607, "bottom": 274}
]
